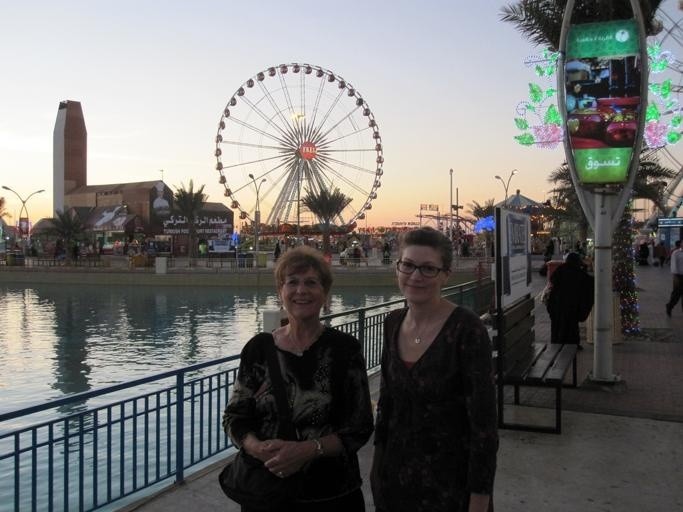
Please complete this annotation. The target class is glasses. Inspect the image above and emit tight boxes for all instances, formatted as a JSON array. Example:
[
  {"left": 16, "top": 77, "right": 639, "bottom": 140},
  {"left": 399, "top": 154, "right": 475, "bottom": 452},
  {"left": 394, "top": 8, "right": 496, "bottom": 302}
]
[{"left": 395, "top": 259, "right": 446, "bottom": 279}]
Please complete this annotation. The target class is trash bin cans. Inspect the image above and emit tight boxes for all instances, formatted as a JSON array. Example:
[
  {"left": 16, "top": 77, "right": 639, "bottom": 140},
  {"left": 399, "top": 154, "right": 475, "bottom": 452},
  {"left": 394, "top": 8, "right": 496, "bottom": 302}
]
[
  {"left": 548, "top": 261, "right": 562, "bottom": 280},
  {"left": 6, "top": 253, "right": 25, "bottom": 266},
  {"left": 239, "top": 254, "right": 253, "bottom": 267},
  {"left": 256, "top": 252, "right": 267, "bottom": 267}
]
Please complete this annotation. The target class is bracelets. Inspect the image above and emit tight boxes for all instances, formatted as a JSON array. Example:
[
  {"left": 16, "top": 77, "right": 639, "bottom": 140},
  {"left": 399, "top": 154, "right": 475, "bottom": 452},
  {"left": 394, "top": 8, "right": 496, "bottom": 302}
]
[{"left": 313, "top": 439, "right": 325, "bottom": 456}]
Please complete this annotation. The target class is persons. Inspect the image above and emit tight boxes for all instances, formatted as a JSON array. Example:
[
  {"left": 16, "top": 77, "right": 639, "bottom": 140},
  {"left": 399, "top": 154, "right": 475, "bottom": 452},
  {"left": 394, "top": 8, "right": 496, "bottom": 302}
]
[
  {"left": 369, "top": 226, "right": 499, "bottom": 512},
  {"left": 537, "top": 239, "right": 594, "bottom": 350},
  {"left": 274, "top": 243, "right": 281, "bottom": 261},
  {"left": 153, "top": 182, "right": 170, "bottom": 215},
  {"left": 222, "top": 245, "right": 374, "bottom": 512},
  {"left": 336, "top": 237, "right": 393, "bottom": 268},
  {"left": 457, "top": 236, "right": 486, "bottom": 258},
  {"left": 636, "top": 231, "right": 683, "bottom": 318}
]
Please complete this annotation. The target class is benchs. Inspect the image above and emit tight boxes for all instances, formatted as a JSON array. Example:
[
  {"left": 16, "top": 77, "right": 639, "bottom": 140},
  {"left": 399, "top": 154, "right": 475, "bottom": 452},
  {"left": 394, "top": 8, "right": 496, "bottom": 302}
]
[
  {"left": 338, "top": 253, "right": 368, "bottom": 268},
  {"left": 498, "top": 293, "right": 579, "bottom": 434},
  {"left": 486, "top": 293, "right": 530, "bottom": 405}
]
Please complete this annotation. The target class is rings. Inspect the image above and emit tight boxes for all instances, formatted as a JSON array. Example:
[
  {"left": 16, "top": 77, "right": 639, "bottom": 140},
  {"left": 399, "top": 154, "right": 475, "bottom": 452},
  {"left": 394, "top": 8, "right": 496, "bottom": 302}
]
[{"left": 280, "top": 472, "right": 285, "bottom": 476}]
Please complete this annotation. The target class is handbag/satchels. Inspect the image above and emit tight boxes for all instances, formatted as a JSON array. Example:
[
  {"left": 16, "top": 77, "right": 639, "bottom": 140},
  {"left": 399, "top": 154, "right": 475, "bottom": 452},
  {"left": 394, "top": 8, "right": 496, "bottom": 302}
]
[{"left": 216, "top": 330, "right": 299, "bottom": 511}]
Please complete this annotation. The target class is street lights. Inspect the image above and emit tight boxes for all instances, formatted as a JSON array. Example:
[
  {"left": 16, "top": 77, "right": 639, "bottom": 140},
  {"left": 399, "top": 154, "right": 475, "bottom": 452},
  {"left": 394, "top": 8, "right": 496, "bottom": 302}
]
[
  {"left": 448, "top": 168, "right": 455, "bottom": 242},
  {"left": 1, "top": 185, "right": 44, "bottom": 248},
  {"left": 494, "top": 169, "right": 517, "bottom": 208},
  {"left": 249, "top": 173, "right": 266, "bottom": 268}
]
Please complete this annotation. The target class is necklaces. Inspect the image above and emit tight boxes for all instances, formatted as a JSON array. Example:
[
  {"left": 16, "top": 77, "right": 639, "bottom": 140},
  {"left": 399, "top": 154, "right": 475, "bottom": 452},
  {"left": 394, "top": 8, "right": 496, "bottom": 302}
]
[
  {"left": 415, "top": 335, "right": 423, "bottom": 345},
  {"left": 288, "top": 335, "right": 317, "bottom": 357}
]
